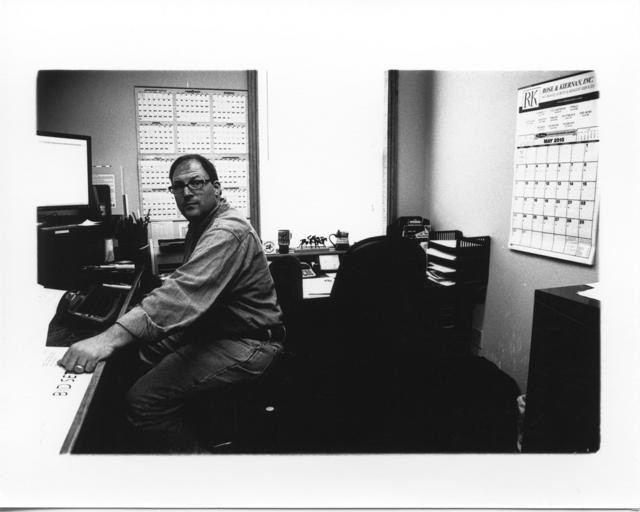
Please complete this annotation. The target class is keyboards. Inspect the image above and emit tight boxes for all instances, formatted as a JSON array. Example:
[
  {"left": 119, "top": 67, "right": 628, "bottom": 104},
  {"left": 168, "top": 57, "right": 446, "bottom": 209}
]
[{"left": 68, "top": 287, "right": 123, "bottom": 327}]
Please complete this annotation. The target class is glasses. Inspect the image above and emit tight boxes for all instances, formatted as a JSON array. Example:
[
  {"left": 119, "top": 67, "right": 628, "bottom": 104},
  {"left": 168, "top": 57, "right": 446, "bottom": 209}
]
[{"left": 169, "top": 178, "right": 209, "bottom": 194}]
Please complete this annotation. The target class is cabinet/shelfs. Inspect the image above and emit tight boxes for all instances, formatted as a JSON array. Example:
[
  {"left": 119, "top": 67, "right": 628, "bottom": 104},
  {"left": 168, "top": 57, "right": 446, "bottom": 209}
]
[
  {"left": 521, "top": 284, "right": 600, "bottom": 452},
  {"left": 427, "top": 230, "right": 491, "bottom": 294}
]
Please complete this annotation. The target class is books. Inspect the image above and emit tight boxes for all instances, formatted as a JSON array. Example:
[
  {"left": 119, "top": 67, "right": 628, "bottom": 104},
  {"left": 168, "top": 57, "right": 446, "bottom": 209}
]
[
  {"left": 308, "top": 277, "right": 335, "bottom": 296},
  {"left": 427, "top": 270, "right": 452, "bottom": 281}
]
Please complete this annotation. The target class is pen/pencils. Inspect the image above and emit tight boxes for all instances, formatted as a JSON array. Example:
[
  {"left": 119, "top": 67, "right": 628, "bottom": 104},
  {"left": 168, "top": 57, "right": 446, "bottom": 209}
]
[{"left": 119, "top": 208, "right": 150, "bottom": 232}]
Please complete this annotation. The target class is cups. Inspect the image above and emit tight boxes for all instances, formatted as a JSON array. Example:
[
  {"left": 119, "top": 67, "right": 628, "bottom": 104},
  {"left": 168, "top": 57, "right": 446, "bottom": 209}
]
[{"left": 329, "top": 232, "right": 350, "bottom": 251}]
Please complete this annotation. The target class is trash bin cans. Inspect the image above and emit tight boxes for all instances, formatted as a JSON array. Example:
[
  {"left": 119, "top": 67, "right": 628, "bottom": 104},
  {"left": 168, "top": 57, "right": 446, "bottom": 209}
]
[{"left": 515, "top": 394, "right": 526, "bottom": 453}]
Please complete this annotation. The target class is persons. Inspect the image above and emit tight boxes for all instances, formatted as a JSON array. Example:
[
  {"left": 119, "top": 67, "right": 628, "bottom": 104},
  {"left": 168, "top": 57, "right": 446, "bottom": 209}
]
[{"left": 57, "top": 153, "right": 287, "bottom": 451}]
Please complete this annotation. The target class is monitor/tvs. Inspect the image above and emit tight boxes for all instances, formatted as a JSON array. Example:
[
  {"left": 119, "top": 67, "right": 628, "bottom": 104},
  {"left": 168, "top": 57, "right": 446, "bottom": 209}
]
[{"left": 36, "top": 131, "right": 93, "bottom": 227}]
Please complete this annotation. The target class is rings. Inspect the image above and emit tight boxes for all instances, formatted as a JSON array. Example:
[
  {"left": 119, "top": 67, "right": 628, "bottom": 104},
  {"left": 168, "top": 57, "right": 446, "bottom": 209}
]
[{"left": 76, "top": 364, "right": 86, "bottom": 368}]
